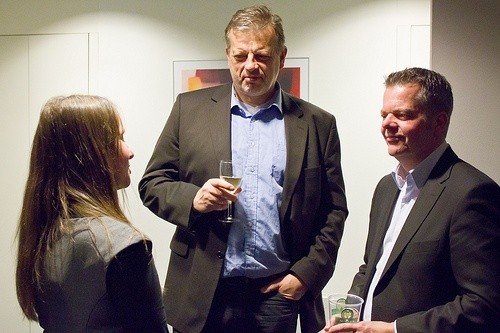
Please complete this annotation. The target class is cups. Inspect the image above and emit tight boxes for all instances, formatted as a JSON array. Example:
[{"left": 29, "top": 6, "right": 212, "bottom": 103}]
[{"left": 328, "top": 294, "right": 364, "bottom": 333}]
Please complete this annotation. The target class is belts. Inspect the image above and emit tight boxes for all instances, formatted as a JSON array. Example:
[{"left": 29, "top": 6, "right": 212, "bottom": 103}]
[{"left": 215, "top": 275, "right": 282, "bottom": 303}]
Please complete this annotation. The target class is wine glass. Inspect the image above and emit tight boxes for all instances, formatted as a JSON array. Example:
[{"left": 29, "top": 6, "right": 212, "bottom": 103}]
[{"left": 218, "top": 159, "right": 244, "bottom": 223}]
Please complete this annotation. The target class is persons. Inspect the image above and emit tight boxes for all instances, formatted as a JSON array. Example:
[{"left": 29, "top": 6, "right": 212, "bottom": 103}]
[
  {"left": 316, "top": 66, "right": 500, "bottom": 333},
  {"left": 138, "top": 3, "right": 350, "bottom": 333},
  {"left": 12, "top": 94, "right": 169, "bottom": 333}
]
